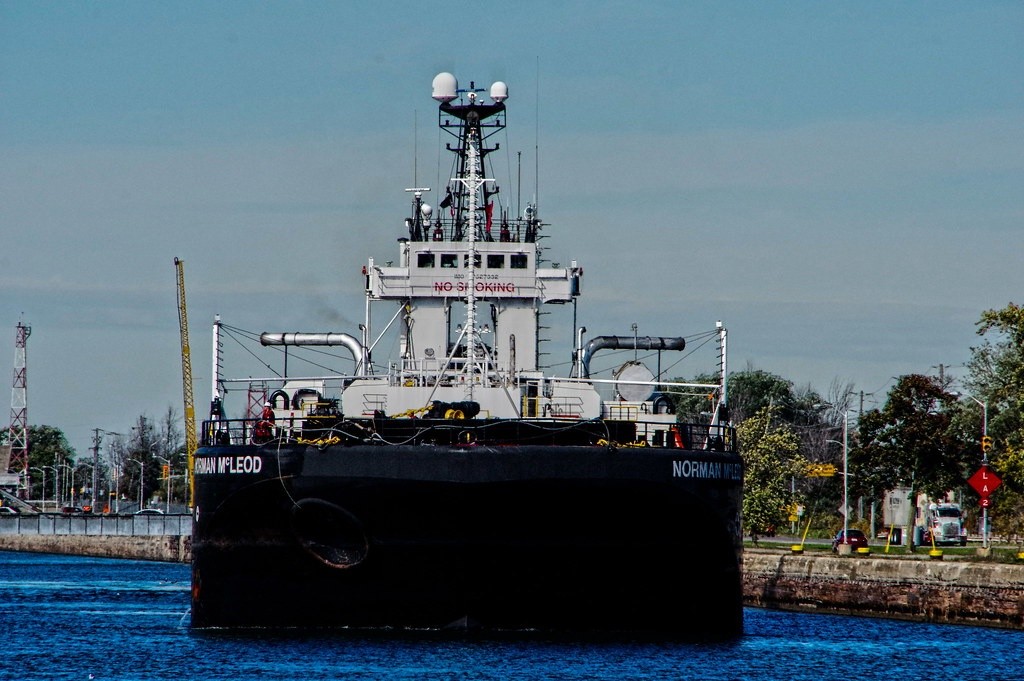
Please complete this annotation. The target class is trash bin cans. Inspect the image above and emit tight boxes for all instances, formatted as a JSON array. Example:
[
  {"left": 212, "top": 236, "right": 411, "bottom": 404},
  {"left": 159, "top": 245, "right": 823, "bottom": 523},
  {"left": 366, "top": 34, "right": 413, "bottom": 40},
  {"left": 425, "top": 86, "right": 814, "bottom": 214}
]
[{"left": 960, "top": 535, "right": 967, "bottom": 546}]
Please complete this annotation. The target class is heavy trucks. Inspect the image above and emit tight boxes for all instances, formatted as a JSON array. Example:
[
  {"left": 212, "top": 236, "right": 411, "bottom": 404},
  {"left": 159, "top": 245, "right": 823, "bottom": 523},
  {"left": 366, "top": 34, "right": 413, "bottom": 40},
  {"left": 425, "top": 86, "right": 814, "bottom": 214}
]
[{"left": 883, "top": 489, "right": 967, "bottom": 548}]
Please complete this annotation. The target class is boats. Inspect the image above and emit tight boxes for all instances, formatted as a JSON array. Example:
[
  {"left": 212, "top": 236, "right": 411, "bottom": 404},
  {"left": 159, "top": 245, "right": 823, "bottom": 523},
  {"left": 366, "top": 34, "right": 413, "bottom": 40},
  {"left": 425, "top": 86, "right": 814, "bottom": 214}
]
[{"left": 186, "top": 72, "right": 746, "bottom": 650}]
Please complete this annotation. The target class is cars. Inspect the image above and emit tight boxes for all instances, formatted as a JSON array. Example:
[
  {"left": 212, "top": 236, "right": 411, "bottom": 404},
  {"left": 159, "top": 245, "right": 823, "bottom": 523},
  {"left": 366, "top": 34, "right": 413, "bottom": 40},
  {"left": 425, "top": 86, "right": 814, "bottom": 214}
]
[
  {"left": 832, "top": 529, "right": 868, "bottom": 551},
  {"left": 0, "top": 505, "right": 19, "bottom": 513},
  {"left": 134, "top": 509, "right": 164, "bottom": 514}
]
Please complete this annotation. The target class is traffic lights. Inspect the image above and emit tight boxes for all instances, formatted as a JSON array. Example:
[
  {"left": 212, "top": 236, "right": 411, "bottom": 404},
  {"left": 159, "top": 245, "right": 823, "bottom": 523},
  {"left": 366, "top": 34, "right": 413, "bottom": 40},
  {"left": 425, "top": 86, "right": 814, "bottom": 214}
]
[
  {"left": 163, "top": 466, "right": 167, "bottom": 478},
  {"left": 983, "top": 436, "right": 992, "bottom": 452}
]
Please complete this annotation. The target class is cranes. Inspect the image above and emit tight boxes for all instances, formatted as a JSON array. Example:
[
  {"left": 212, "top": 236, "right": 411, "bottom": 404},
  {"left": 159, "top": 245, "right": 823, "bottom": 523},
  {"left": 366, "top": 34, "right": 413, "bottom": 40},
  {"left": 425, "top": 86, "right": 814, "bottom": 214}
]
[{"left": 173, "top": 253, "right": 195, "bottom": 513}]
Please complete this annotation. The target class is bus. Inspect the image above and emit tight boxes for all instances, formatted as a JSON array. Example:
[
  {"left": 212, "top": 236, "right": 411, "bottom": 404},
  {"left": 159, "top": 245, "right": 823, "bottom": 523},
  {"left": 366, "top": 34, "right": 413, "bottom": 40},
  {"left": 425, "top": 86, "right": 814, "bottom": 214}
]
[{"left": 24, "top": 500, "right": 62, "bottom": 512}]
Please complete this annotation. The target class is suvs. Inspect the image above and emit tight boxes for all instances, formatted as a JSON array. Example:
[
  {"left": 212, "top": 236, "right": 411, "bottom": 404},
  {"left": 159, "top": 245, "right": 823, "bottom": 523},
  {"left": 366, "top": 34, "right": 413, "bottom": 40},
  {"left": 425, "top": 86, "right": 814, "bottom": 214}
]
[{"left": 63, "top": 506, "right": 82, "bottom": 513}]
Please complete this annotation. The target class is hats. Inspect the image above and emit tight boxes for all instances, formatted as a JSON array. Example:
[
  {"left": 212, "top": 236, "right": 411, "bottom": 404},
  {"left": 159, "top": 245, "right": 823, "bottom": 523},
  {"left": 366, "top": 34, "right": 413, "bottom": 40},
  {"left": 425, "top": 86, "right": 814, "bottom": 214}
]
[{"left": 264, "top": 401, "right": 271, "bottom": 406}]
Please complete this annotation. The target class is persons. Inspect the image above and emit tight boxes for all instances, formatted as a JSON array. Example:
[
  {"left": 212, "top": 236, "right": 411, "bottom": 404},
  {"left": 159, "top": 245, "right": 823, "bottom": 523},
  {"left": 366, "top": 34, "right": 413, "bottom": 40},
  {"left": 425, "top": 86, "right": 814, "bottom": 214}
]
[{"left": 253, "top": 402, "right": 275, "bottom": 445}]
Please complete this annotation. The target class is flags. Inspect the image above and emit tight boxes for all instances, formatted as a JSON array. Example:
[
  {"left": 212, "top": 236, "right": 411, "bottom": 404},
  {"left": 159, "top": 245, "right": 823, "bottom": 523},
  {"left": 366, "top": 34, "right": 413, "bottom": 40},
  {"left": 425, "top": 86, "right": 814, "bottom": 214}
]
[
  {"left": 485, "top": 201, "right": 493, "bottom": 231},
  {"left": 440, "top": 187, "right": 455, "bottom": 217}
]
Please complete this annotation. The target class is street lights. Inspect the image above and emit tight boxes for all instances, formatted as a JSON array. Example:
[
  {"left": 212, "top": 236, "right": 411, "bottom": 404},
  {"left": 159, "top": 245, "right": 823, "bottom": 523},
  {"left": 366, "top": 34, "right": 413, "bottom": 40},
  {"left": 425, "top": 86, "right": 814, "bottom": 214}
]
[
  {"left": 127, "top": 458, "right": 143, "bottom": 510},
  {"left": 812, "top": 402, "right": 847, "bottom": 543},
  {"left": 29, "top": 467, "right": 44, "bottom": 512},
  {"left": 101, "top": 456, "right": 119, "bottom": 513},
  {"left": 152, "top": 455, "right": 170, "bottom": 515},
  {"left": 42, "top": 465, "right": 58, "bottom": 511},
  {"left": 78, "top": 462, "right": 96, "bottom": 514},
  {"left": 57, "top": 464, "right": 74, "bottom": 507},
  {"left": 951, "top": 391, "right": 987, "bottom": 549}
]
